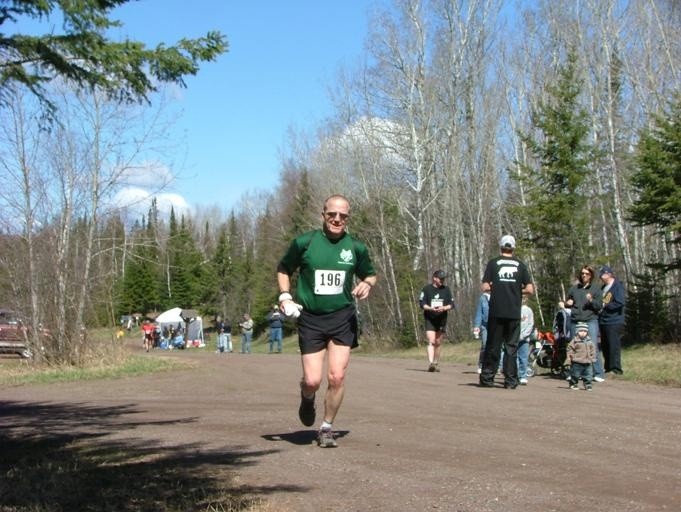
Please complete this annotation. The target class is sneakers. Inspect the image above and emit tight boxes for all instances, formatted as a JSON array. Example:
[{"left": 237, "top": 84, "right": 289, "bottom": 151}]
[
  {"left": 299, "top": 390, "right": 315, "bottom": 426},
  {"left": 478, "top": 367, "right": 614, "bottom": 391},
  {"left": 316, "top": 427, "right": 337, "bottom": 448},
  {"left": 429, "top": 363, "right": 440, "bottom": 372}
]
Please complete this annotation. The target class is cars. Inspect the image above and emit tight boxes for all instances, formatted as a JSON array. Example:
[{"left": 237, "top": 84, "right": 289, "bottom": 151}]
[{"left": 116, "top": 315, "right": 134, "bottom": 326}]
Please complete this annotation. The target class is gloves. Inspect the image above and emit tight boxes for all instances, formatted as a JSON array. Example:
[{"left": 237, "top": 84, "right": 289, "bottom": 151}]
[{"left": 284, "top": 303, "right": 303, "bottom": 318}]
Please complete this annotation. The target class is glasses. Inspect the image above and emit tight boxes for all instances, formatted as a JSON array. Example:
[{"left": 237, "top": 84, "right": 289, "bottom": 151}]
[
  {"left": 581, "top": 272, "right": 589, "bottom": 275},
  {"left": 327, "top": 213, "right": 349, "bottom": 220}
]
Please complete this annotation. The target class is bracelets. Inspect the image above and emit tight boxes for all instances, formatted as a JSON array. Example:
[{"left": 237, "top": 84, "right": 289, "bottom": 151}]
[
  {"left": 279, "top": 291, "right": 290, "bottom": 295},
  {"left": 361, "top": 280, "right": 373, "bottom": 289}
]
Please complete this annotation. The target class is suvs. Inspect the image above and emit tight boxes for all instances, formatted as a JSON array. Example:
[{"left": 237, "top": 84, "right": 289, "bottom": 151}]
[{"left": 0, "top": 310, "right": 51, "bottom": 358}]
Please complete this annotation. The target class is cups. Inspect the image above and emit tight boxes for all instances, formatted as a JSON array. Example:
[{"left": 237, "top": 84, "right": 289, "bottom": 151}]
[
  {"left": 474, "top": 330, "right": 480, "bottom": 339},
  {"left": 564, "top": 370, "right": 572, "bottom": 381}
]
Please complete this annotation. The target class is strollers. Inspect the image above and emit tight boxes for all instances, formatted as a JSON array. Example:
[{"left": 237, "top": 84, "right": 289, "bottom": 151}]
[{"left": 524, "top": 310, "right": 574, "bottom": 378}]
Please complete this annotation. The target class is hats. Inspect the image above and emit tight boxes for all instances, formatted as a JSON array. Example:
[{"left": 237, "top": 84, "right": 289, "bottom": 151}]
[
  {"left": 434, "top": 270, "right": 447, "bottom": 279},
  {"left": 500, "top": 234, "right": 515, "bottom": 249},
  {"left": 599, "top": 266, "right": 612, "bottom": 277}
]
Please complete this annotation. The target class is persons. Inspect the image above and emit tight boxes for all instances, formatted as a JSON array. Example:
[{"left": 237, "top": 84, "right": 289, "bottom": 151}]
[
  {"left": 473, "top": 232, "right": 626, "bottom": 391},
  {"left": 274, "top": 193, "right": 378, "bottom": 451},
  {"left": 113, "top": 302, "right": 287, "bottom": 353},
  {"left": 418, "top": 268, "right": 454, "bottom": 372}
]
